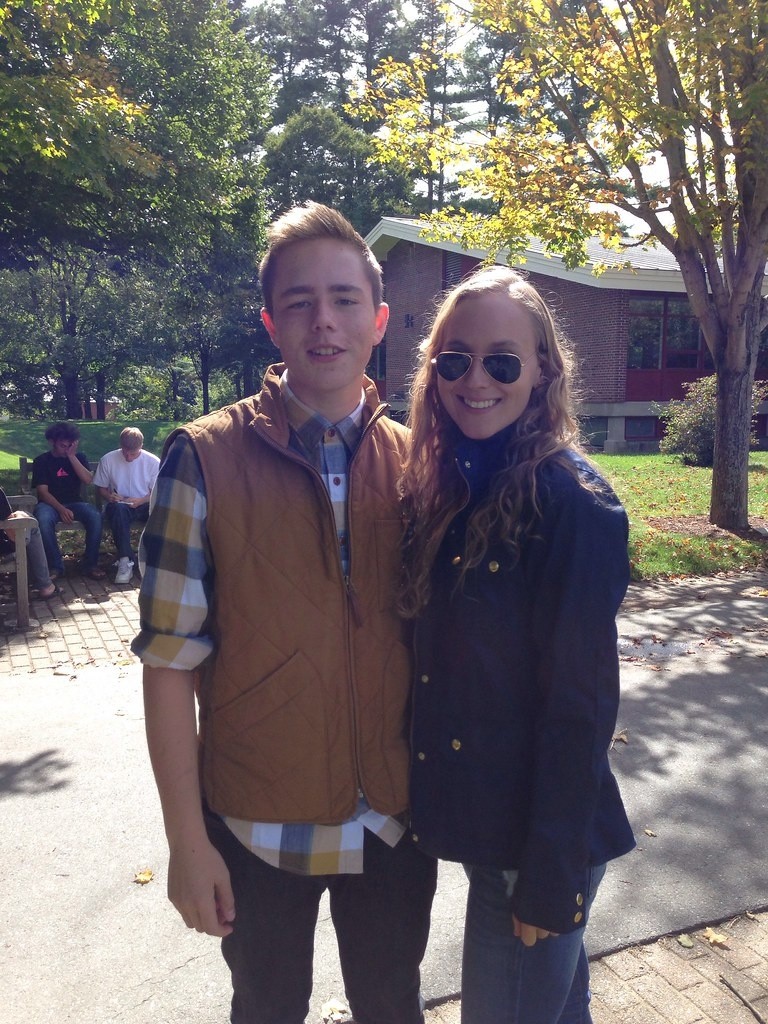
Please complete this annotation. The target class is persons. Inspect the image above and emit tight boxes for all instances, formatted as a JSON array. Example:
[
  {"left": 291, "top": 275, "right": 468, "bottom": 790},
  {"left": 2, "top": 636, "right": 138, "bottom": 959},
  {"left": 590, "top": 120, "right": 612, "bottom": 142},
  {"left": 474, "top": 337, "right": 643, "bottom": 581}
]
[
  {"left": 392, "top": 268, "right": 638, "bottom": 1024},
  {"left": 94, "top": 427, "right": 161, "bottom": 583},
  {"left": 31, "top": 421, "right": 108, "bottom": 580},
  {"left": 132, "top": 199, "right": 438, "bottom": 1024},
  {"left": 0, "top": 487, "right": 66, "bottom": 601}
]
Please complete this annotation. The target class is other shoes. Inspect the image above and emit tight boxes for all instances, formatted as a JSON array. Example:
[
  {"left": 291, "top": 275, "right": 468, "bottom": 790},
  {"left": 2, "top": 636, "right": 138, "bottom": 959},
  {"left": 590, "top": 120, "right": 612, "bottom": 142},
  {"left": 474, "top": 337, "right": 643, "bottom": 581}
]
[{"left": 84, "top": 568, "right": 105, "bottom": 580}]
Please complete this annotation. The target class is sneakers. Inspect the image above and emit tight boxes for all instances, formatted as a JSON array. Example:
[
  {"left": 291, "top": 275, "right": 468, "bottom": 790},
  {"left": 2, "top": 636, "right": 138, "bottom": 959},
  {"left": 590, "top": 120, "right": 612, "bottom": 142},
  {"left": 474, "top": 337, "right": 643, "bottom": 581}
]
[{"left": 114, "top": 560, "right": 134, "bottom": 584}]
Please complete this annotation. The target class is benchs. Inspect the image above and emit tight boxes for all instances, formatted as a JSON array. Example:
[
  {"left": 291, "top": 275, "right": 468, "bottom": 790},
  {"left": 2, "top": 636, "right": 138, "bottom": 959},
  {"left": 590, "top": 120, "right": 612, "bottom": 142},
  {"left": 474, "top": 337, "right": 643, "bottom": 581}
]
[{"left": 19, "top": 457, "right": 145, "bottom": 530}]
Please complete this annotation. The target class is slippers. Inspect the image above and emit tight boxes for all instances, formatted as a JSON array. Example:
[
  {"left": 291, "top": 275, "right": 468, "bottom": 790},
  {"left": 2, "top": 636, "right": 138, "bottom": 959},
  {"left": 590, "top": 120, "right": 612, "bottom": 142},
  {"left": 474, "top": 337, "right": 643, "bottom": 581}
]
[{"left": 40, "top": 584, "right": 65, "bottom": 600}]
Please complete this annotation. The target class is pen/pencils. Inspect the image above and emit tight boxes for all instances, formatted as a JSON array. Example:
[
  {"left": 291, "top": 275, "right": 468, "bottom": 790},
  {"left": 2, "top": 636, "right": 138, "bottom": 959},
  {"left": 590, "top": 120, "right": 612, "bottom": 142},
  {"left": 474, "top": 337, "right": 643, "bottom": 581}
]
[{"left": 114, "top": 488, "right": 121, "bottom": 502}]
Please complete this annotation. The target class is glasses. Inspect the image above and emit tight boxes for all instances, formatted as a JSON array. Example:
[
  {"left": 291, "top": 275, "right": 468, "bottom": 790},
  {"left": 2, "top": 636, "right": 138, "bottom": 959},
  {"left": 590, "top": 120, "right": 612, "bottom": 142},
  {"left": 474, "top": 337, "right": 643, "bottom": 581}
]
[{"left": 431, "top": 349, "right": 539, "bottom": 384}]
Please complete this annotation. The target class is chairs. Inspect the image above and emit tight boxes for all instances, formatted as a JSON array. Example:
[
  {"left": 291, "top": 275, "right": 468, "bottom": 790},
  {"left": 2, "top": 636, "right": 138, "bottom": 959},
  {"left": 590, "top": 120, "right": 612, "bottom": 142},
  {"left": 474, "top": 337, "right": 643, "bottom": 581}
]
[{"left": 0, "top": 495, "right": 39, "bottom": 631}]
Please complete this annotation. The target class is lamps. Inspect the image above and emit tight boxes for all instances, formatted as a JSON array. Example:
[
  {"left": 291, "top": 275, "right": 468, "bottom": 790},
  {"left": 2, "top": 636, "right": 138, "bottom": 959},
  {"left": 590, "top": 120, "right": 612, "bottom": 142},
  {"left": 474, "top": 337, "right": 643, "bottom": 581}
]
[{"left": 404, "top": 314, "right": 414, "bottom": 328}]
